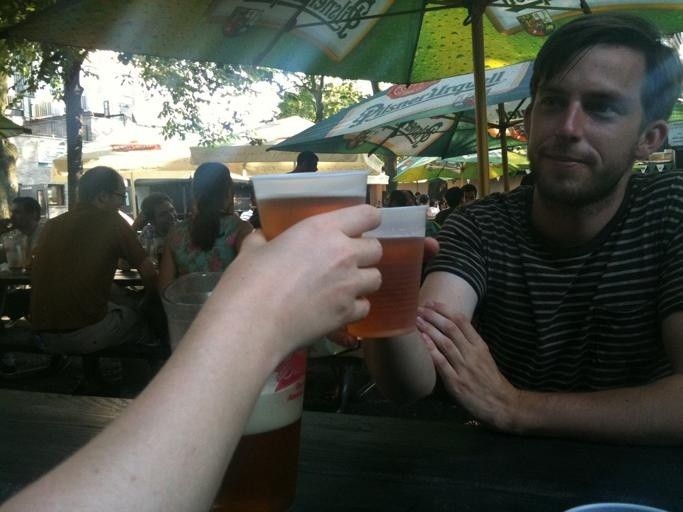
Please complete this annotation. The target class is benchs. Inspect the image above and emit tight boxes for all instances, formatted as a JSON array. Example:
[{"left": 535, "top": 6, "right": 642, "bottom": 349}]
[{"left": 0, "top": 326, "right": 169, "bottom": 381}]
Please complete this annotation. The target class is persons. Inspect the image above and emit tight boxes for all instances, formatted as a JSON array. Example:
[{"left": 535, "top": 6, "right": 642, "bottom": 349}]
[
  {"left": 0, "top": 202, "right": 383, "bottom": 512},
  {"left": 0, "top": 150, "right": 482, "bottom": 411},
  {"left": 355, "top": 14, "right": 683, "bottom": 463}
]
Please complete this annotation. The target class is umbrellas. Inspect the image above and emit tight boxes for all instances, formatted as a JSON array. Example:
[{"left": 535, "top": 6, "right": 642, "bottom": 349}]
[
  {"left": 265, "top": 47, "right": 682, "bottom": 197},
  {"left": 46, "top": 119, "right": 205, "bottom": 225},
  {"left": 188, "top": 113, "right": 384, "bottom": 178},
  {"left": 0, "top": 0, "right": 683, "bottom": 209}
]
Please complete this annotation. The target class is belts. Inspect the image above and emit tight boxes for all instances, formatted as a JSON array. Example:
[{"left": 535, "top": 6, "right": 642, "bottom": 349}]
[{"left": 42, "top": 329, "right": 79, "bottom": 334}]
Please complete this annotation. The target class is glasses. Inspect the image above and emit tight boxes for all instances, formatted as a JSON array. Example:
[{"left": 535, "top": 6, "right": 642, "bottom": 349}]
[{"left": 113, "top": 191, "right": 128, "bottom": 199}]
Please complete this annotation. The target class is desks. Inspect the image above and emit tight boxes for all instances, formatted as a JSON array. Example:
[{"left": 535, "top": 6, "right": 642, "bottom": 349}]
[{"left": 1, "top": 264, "right": 163, "bottom": 373}]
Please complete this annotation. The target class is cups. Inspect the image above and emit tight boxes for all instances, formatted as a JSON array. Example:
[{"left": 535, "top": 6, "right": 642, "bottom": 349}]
[
  {"left": 345, "top": 204, "right": 429, "bottom": 339},
  {"left": 159, "top": 271, "right": 310, "bottom": 512},
  {"left": 253, "top": 168, "right": 369, "bottom": 242}
]
